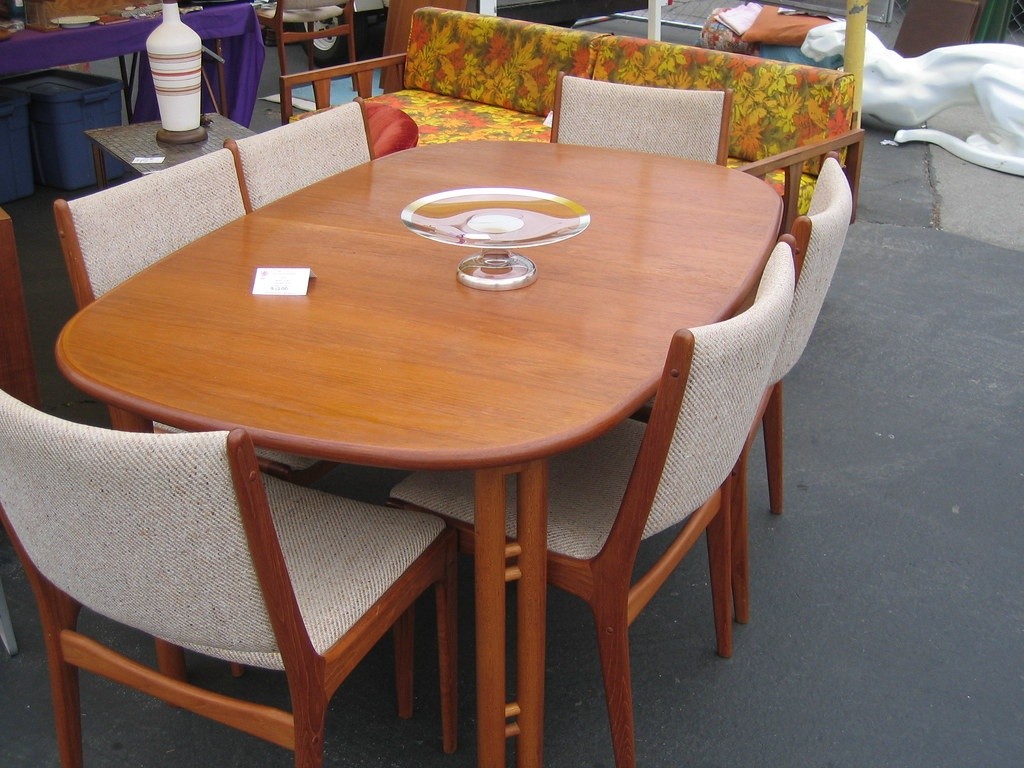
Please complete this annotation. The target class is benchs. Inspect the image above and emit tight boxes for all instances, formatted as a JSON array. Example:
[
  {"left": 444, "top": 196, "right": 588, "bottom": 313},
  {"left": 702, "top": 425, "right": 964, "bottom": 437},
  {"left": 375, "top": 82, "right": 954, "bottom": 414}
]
[{"left": 274, "top": 3, "right": 863, "bottom": 247}]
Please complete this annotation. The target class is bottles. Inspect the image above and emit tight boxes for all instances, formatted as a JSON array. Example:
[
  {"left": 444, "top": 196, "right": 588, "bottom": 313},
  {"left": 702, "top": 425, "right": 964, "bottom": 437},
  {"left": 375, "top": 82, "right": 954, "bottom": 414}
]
[{"left": 146, "top": 3, "right": 201, "bottom": 131}]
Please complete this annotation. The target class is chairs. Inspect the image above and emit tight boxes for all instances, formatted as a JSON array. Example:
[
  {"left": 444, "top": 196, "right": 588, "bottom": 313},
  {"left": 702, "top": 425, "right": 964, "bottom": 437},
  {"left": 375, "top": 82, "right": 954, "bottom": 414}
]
[
  {"left": 53, "top": 147, "right": 346, "bottom": 489},
  {"left": 547, "top": 72, "right": 734, "bottom": 165},
  {"left": 251, "top": 0, "right": 359, "bottom": 108},
  {"left": 621, "top": 149, "right": 855, "bottom": 517},
  {"left": 387, "top": 234, "right": 802, "bottom": 766},
  {"left": 0, "top": 390, "right": 461, "bottom": 767},
  {"left": 220, "top": 99, "right": 371, "bottom": 217}
]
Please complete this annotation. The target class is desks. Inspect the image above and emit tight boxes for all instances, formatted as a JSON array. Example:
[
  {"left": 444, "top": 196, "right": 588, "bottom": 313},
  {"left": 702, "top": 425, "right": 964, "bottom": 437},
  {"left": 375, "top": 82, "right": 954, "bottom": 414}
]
[
  {"left": 0, "top": 0, "right": 267, "bottom": 130},
  {"left": 53, "top": 133, "right": 789, "bottom": 768},
  {"left": 86, "top": 112, "right": 259, "bottom": 198}
]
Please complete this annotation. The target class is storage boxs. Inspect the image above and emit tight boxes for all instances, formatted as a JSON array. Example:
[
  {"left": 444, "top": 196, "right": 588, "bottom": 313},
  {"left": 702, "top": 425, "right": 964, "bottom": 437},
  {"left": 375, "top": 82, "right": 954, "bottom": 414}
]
[
  {"left": 2, "top": 67, "right": 129, "bottom": 191},
  {"left": 0, "top": 86, "right": 35, "bottom": 206}
]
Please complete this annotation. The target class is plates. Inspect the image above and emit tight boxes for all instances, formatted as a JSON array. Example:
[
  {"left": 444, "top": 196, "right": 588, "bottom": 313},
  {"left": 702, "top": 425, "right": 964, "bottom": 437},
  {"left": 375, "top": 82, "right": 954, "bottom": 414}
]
[{"left": 51, "top": 16, "right": 100, "bottom": 28}]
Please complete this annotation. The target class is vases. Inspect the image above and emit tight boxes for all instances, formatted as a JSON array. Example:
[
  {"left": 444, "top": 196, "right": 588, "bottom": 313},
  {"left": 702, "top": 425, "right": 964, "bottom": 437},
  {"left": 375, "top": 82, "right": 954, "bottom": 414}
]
[{"left": 145, "top": 1, "right": 210, "bottom": 147}]
